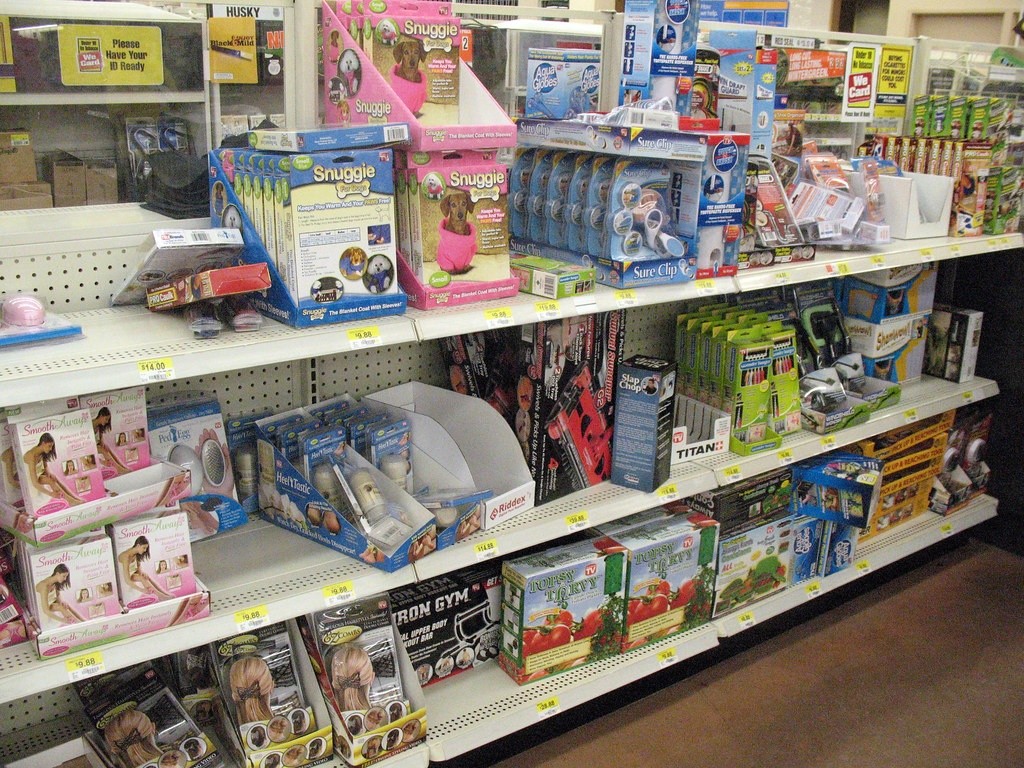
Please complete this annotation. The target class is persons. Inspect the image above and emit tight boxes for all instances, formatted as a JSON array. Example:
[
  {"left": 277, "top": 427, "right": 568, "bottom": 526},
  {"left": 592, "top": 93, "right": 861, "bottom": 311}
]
[
  {"left": 92, "top": 406, "right": 133, "bottom": 478},
  {"left": 24, "top": 431, "right": 86, "bottom": 512},
  {"left": 118, "top": 535, "right": 176, "bottom": 604},
  {"left": 34, "top": 561, "right": 92, "bottom": 630}
]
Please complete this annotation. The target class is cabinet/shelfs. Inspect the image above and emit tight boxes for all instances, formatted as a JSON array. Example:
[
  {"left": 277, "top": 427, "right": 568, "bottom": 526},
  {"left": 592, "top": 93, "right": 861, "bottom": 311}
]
[{"left": 0, "top": 0, "right": 1024, "bottom": 768}]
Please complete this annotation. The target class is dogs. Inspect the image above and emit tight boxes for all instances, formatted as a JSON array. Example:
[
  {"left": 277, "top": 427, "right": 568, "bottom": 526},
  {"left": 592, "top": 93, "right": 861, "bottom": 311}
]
[
  {"left": 379, "top": 20, "right": 399, "bottom": 46},
  {"left": 367, "top": 255, "right": 391, "bottom": 294},
  {"left": 340, "top": 50, "right": 359, "bottom": 96},
  {"left": 338, "top": 247, "right": 366, "bottom": 277},
  {"left": 328, "top": 31, "right": 340, "bottom": 62},
  {"left": 439, "top": 193, "right": 477, "bottom": 275},
  {"left": 335, "top": 100, "right": 350, "bottom": 124},
  {"left": 426, "top": 174, "right": 443, "bottom": 200},
  {"left": 392, "top": 41, "right": 427, "bottom": 119},
  {"left": 214, "top": 182, "right": 224, "bottom": 215},
  {"left": 225, "top": 206, "right": 242, "bottom": 233}
]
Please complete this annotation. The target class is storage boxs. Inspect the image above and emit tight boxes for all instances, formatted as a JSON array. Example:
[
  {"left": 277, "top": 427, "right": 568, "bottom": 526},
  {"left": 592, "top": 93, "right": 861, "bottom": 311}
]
[{"left": 0, "top": 0, "right": 1024, "bottom": 768}]
